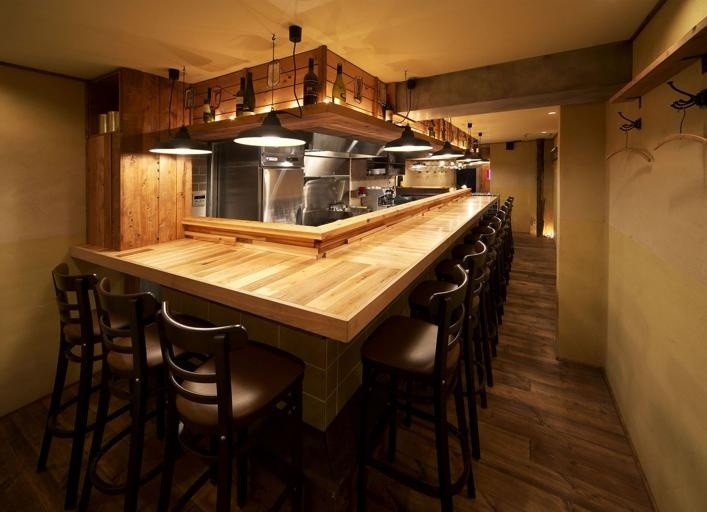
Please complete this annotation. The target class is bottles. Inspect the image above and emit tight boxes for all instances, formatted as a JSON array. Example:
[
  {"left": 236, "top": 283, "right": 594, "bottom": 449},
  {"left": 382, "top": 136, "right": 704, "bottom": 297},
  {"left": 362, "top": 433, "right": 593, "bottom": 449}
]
[
  {"left": 332, "top": 62, "right": 346, "bottom": 106},
  {"left": 303, "top": 59, "right": 319, "bottom": 106},
  {"left": 202, "top": 87, "right": 215, "bottom": 123},
  {"left": 236, "top": 71, "right": 256, "bottom": 118},
  {"left": 384, "top": 95, "right": 392, "bottom": 123}
]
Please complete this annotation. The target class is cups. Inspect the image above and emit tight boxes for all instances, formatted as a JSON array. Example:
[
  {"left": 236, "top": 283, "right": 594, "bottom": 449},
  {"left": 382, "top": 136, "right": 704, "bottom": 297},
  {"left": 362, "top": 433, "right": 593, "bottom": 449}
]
[{"left": 99, "top": 111, "right": 120, "bottom": 134}]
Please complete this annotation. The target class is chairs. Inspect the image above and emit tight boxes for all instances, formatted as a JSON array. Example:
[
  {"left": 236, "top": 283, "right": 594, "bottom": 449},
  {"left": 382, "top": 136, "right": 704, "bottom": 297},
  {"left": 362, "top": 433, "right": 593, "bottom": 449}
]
[
  {"left": 80, "top": 278, "right": 184, "bottom": 512},
  {"left": 356, "top": 195, "right": 516, "bottom": 512},
  {"left": 157, "top": 300, "right": 306, "bottom": 512},
  {"left": 37, "top": 262, "right": 130, "bottom": 512}
]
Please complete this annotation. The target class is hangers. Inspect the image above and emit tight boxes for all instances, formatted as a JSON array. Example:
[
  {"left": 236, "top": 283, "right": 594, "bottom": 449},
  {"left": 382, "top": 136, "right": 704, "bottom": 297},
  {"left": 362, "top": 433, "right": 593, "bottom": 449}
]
[
  {"left": 606, "top": 129, "right": 651, "bottom": 162},
  {"left": 654, "top": 106, "right": 707, "bottom": 151}
]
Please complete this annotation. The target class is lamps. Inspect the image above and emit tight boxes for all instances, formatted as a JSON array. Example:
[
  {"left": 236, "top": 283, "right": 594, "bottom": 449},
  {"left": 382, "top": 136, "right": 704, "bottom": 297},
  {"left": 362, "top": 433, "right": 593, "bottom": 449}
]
[
  {"left": 456, "top": 123, "right": 481, "bottom": 161},
  {"left": 383, "top": 70, "right": 433, "bottom": 153},
  {"left": 432, "top": 118, "right": 464, "bottom": 157},
  {"left": 148, "top": 68, "right": 213, "bottom": 155},
  {"left": 471, "top": 132, "right": 490, "bottom": 163},
  {"left": 233, "top": 25, "right": 307, "bottom": 148}
]
